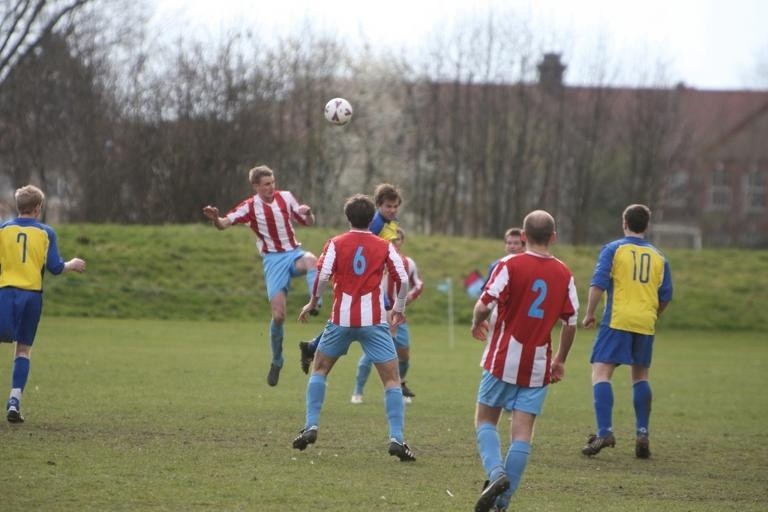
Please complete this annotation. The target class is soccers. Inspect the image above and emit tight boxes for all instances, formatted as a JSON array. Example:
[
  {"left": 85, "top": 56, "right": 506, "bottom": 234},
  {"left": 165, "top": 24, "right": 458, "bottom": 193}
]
[{"left": 325, "top": 98, "right": 352, "bottom": 125}]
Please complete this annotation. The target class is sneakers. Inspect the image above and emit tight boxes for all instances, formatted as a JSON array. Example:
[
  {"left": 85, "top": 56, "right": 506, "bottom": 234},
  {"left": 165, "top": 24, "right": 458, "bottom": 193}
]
[
  {"left": 298, "top": 341, "right": 313, "bottom": 375},
  {"left": 5, "top": 397, "right": 25, "bottom": 422},
  {"left": 401, "top": 382, "right": 415, "bottom": 398},
  {"left": 350, "top": 391, "right": 364, "bottom": 404},
  {"left": 267, "top": 357, "right": 284, "bottom": 387},
  {"left": 635, "top": 437, "right": 653, "bottom": 459},
  {"left": 388, "top": 438, "right": 416, "bottom": 461},
  {"left": 310, "top": 296, "right": 322, "bottom": 315},
  {"left": 580, "top": 431, "right": 617, "bottom": 457},
  {"left": 292, "top": 428, "right": 317, "bottom": 450},
  {"left": 472, "top": 474, "right": 511, "bottom": 512}
]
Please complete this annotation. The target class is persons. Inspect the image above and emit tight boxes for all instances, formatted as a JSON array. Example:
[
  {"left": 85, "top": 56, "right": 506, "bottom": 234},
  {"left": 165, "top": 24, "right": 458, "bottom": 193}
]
[
  {"left": 293, "top": 194, "right": 416, "bottom": 460},
  {"left": 471, "top": 209, "right": 579, "bottom": 511},
  {"left": 351, "top": 227, "right": 416, "bottom": 404},
  {"left": 203, "top": 165, "right": 323, "bottom": 387},
  {"left": 481, "top": 228, "right": 526, "bottom": 293},
  {"left": 298, "top": 183, "right": 401, "bottom": 374},
  {"left": 582, "top": 205, "right": 672, "bottom": 459},
  {"left": 1, "top": 183, "right": 86, "bottom": 424}
]
